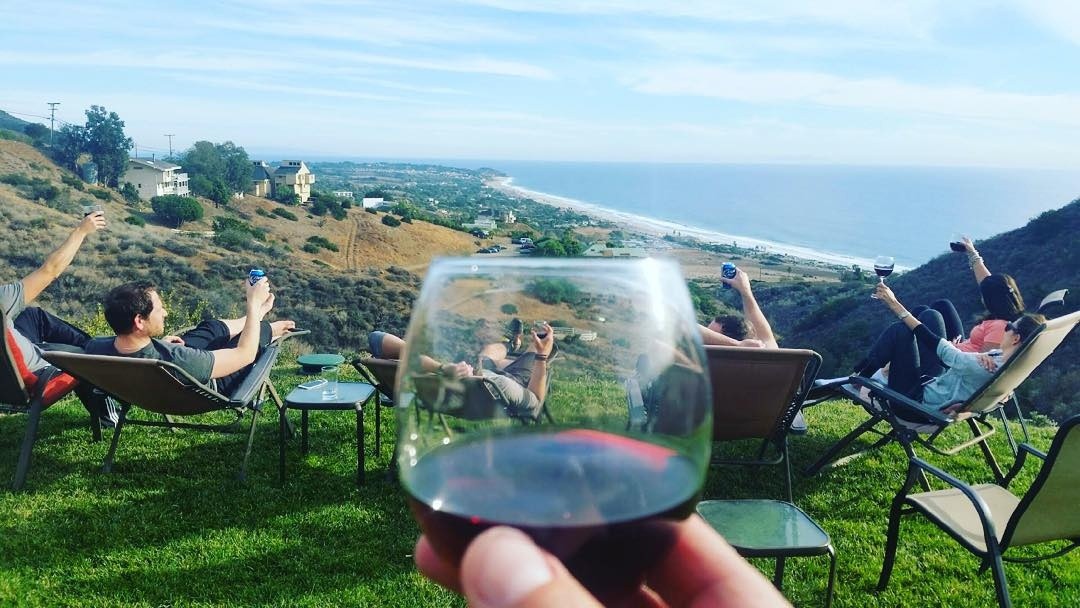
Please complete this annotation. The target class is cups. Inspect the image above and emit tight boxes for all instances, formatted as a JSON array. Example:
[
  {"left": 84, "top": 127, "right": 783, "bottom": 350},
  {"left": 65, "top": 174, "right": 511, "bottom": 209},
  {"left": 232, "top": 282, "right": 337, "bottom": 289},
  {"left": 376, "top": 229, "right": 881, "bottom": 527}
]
[
  {"left": 948, "top": 232, "right": 969, "bottom": 252},
  {"left": 393, "top": 257, "right": 714, "bottom": 605},
  {"left": 84, "top": 205, "right": 104, "bottom": 232},
  {"left": 534, "top": 321, "right": 548, "bottom": 339},
  {"left": 321, "top": 366, "right": 338, "bottom": 400}
]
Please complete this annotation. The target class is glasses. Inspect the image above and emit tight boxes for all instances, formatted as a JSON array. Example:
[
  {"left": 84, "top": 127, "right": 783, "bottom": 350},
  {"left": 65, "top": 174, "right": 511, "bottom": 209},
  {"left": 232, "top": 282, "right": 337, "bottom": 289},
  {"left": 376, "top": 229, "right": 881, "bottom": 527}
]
[{"left": 1005, "top": 321, "right": 1019, "bottom": 333}]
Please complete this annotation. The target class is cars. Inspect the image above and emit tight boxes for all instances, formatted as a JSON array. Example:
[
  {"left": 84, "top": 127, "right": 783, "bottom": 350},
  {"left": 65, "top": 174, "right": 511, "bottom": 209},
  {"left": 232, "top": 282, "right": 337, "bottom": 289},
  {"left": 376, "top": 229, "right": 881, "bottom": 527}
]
[{"left": 473, "top": 230, "right": 533, "bottom": 254}]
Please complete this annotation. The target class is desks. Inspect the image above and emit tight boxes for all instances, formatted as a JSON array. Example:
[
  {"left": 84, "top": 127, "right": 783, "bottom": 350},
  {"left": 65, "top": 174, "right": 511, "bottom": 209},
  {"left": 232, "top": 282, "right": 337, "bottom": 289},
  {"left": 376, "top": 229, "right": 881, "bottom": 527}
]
[
  {"left": 279, "top": 382, "right": 383, "bottom": 483},
  {"left": 693, "top": 498, "right": 837, "bottom": 608}
]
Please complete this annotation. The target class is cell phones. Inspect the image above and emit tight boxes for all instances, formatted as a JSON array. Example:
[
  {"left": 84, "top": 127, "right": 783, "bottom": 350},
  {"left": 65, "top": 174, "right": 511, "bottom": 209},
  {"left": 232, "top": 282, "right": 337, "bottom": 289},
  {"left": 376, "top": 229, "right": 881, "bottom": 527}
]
[{"left": 297, "top": 379, "right": 328, "bottom": 390}]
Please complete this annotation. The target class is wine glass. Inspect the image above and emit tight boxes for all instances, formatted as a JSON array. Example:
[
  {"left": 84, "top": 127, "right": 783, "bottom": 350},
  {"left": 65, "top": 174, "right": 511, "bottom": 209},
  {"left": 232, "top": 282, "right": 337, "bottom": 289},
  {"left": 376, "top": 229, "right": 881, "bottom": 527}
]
[{"left": 870, "top": 257, "right": 895, "bottom": 299}]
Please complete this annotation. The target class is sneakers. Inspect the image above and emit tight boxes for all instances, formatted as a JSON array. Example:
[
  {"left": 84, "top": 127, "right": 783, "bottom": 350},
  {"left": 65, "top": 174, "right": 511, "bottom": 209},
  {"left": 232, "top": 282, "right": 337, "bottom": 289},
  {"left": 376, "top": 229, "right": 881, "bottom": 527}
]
[
  {"left": 815, "top": 375, "right": 860, "bottom": 396},
  {"left": 870, "top": 368, "right": 888, "bottom": 385}
]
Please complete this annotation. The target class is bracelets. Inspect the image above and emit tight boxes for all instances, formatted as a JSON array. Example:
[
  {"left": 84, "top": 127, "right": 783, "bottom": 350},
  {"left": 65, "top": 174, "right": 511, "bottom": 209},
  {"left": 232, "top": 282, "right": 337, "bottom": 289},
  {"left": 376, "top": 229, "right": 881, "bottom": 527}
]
[
  {"left": 968, "top": 250, "right": 983, "bottom": 269},
  {"left": 898, "top": 311, "right": 910, "bottom": 320}
]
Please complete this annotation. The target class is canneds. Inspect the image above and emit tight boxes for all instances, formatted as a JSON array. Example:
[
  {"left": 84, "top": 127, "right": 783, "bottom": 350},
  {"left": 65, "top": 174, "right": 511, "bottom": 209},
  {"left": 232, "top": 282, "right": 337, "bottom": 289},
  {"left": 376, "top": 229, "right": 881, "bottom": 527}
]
[
  {"left": 720, "top": 261, "right": 736, "bottom": 290},
  {"left": 248, "top": 270, "right": 265, "bottom": 286}
]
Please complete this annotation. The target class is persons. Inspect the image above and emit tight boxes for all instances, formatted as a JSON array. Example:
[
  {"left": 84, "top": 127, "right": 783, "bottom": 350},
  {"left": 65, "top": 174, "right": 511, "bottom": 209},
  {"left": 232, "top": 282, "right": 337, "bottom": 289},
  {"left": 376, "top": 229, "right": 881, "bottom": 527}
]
[
  {"left": 85, "top": 277, "right": 296, "bottom": 398},
  {"left": 645, "top": 266, "right": 780, "bottom": 384},
  {"left": 0, "top": 209, "right": 106, "bottom": 384},
  {"left": 417, "top": 431, "right": 795, "bottom": 608},
  {"left": 910, "top": 235, "right": 1023, "bottom": 352},
  {"left": 836, "top": 282, "right": 1046, "bottom": 422},
  {"left": 368, "top": 317, "right": 554, "bottom": 421}
]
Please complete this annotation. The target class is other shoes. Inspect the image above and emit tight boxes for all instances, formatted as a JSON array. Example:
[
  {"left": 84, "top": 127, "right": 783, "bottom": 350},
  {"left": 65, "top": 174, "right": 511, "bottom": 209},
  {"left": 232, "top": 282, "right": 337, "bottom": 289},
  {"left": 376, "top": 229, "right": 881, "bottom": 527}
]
[
  {"left": 636, "top": 355, "right": 650, "bottom": 376},
  {"left": 504, "top": 318, "right": 523, "bottom": 351},
  {"left": 547, "top": 343, "right": 559, "bottom": 364}
]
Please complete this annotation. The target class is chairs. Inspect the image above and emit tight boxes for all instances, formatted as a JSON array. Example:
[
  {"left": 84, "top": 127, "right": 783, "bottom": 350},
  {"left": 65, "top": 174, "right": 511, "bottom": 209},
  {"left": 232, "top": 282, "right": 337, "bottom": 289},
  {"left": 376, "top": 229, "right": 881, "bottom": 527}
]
[
  {"left": 622, "top": 345, "right": 825, "bottom": 503},
  {"left": 43, "top": 326, "right": 311, "bottom": 477},
  {"left": 877, "top": 413, "right": 1080, "bottom": 608},
  {"left": 802, "top": 310, "right": 1080, "bottom": 491},
  {"left": 0, "top": 308, "right": 103, "bottom": 491},
  {"left": 350, "top": 341, "right": 562, "bottom": 463},
  {"left": 981, "top": 288, "right": 1069, "bottom": 454}
]
